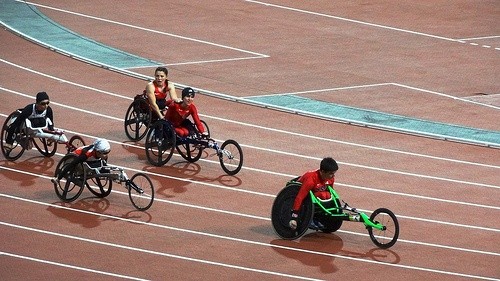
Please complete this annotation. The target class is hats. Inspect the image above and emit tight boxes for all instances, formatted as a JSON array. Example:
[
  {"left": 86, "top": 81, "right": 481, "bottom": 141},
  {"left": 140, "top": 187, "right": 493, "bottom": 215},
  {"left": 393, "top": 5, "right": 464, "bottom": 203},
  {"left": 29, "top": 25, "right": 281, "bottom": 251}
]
[
  {"left": 182, "top": 88, "right": 194, "bottom": 94},
  {"left": 94, "top": 139, "right": 110, "bottom": 151},
  {"left": 36, "top": 91, "right": 49, "bottom": 103}
]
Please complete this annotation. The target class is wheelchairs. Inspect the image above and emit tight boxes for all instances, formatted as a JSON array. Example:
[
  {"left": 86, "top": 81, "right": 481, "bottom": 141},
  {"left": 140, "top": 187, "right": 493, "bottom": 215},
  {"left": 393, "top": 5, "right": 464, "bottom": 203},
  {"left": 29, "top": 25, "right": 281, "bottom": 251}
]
[
  {"left": 124, "top": 90, "right": 244, "bottom": 175},
  {"left": 0, "top": 110, "right": 86, "bottom": 162},
  {"left": 270, "top": 175, "right": 400, "bottom": 249},
  {"left": 50, "top": 152, "right": 154, "bottom": 211}
]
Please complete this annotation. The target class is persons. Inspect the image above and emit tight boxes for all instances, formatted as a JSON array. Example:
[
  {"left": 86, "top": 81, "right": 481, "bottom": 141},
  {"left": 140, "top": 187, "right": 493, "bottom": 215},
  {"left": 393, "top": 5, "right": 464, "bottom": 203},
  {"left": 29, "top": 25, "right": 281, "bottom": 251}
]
[
  {"left": 52, "top": 139, "right": 111, "bottom": 184},
  {"left": 6, "top": 92, "right": 54, "bottom": 153},
  {"left": 289, "top": 156, "right": 340, "bottom": 230},
  {"left": 141, "top": 67, "right": 177, "bottom": 120},
  {"left": 165, "top": 87, "right": 209, "bottom": 138}
]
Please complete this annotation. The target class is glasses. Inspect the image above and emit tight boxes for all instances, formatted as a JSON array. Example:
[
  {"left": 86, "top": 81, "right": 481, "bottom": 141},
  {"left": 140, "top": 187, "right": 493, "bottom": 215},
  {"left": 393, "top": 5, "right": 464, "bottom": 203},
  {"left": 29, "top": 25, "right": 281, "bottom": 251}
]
[
  {"left": 186, "top": 95, "right": 193, "bottom": 98},
  {"left": 103, "top": 150, "right": 111, "bottom": 154},
  {"left": 39, "top": 102, "right": 49, "bottom": 105}
]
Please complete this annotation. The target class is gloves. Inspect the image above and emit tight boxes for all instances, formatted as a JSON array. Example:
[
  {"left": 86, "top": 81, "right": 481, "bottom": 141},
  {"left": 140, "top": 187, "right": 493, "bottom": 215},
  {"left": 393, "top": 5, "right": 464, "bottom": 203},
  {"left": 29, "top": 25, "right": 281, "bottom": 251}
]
[
  {"left": 6, "top": 138, "right": 13, "bottom": 144},
  {"left": 289, "top": 219, "right": 297, "bottom": 229}
]
